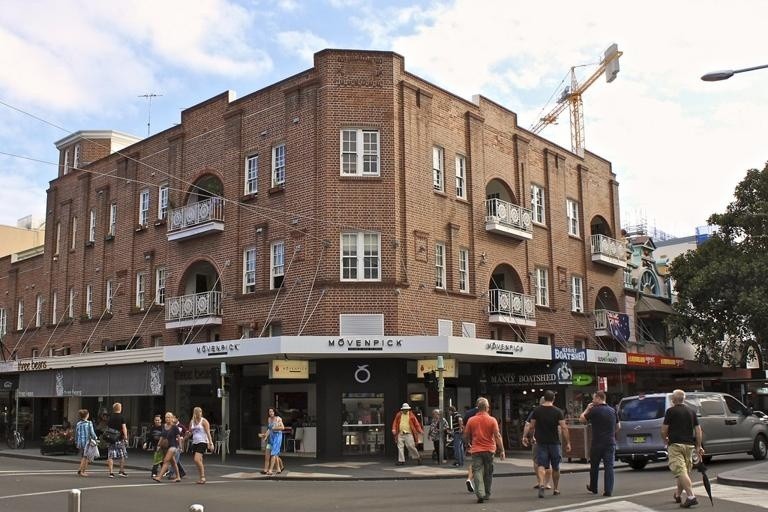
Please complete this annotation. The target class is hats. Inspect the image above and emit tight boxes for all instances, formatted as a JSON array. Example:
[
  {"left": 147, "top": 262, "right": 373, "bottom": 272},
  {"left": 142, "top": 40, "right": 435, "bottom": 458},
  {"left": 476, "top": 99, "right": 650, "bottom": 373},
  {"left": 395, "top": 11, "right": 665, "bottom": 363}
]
[{"left": 401, "top": 403, "right": 411, "bottom": 409}]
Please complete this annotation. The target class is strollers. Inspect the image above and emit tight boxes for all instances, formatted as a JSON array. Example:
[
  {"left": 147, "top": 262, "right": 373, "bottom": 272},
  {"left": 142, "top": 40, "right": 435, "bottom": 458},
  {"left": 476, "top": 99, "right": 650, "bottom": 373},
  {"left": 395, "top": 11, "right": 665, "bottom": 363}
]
[{"left": 430, "top": 432, "right": 454, "bottom": 459}]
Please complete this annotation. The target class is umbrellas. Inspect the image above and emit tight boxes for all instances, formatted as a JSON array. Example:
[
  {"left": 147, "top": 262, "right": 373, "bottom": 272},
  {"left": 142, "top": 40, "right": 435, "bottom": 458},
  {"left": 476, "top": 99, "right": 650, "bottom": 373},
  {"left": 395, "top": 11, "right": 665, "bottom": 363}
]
[{"left": 693, "top": 446, "right": 715, "bottom": 507}]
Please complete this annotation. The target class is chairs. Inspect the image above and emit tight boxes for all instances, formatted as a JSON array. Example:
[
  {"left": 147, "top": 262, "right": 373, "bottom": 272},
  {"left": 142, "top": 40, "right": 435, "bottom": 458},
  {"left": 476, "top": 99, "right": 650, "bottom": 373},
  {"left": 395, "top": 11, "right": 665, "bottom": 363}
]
[
  {"left": 128, "top": 425, "right": 231, "bottom": 455},
  {"left": 286, "top": 427, "right": 306, "bottom": 453}
]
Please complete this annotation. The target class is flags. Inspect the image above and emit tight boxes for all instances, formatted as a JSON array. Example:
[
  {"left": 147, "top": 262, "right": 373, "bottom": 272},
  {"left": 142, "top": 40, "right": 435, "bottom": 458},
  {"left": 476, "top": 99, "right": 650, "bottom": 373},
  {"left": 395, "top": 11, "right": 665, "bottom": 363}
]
[{"left": 606, "top": 313, "right": 630, "bottom": 342}]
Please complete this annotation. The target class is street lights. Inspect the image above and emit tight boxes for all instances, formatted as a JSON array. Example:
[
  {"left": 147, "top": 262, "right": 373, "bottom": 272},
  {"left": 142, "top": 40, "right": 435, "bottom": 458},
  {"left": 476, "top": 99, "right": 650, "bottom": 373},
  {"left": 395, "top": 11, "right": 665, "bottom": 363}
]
[
  {"left": 136, "top": 92, "right": 164, "bottom": 137},
  {"left": 218, "top": 361, "right": 228, "bottom": 462},
  {"left": 435, "top": 355, "right": 446, "bottom": 466},
  {"left": 700, "top": 64, "right": 768, "bottom": 81}
]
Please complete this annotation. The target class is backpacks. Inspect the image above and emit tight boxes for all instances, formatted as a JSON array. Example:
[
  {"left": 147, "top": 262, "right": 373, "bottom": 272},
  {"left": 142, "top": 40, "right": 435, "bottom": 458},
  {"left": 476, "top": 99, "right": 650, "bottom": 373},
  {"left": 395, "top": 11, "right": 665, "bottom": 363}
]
[
  {"left": 103, "top": 428, "right": 121, "bottom": 442},
  {"left": 89, "top": 438, "right": 96, "bottom": 447}
]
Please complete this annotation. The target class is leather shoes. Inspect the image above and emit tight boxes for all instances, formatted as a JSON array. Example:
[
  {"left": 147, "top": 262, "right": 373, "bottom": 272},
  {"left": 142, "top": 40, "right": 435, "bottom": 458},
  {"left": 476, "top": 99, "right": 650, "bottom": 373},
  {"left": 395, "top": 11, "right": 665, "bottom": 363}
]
[{"left": 587, "top": 485, "right": 597, "bottom": 493}]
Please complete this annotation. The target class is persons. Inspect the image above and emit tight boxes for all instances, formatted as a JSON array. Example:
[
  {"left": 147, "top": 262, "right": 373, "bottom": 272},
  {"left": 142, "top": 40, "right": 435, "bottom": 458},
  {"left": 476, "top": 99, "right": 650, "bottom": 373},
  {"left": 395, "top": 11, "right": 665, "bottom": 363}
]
[
  {"left": 391, "top": 402, "right": 425, "bottom": 466},
  {"left": 345, "top": 402, "right": 371, "bottom": 456},
  {"left": 150, "top": 407, "right": 214, "bottom": 483},
  {"left": 463, "top": 389, "right": 623, "bottom": 508},
  {"left": 107, "top": 403, "right": 129, "bottom": 477},
  {"left": 263, "top": 409, "right": 285, "bottom": 475},
  {"left": 660, "top": 387, "right": 707, "bottom": 508},
  {"left": 447, "top": 405, "right": 466, "bottom": 467},
  {"left": 75, "top": 409, "right": 97, "bottom": 477},
  {"left": 428, "top": 409, "right": 451, "bottom": 465}
]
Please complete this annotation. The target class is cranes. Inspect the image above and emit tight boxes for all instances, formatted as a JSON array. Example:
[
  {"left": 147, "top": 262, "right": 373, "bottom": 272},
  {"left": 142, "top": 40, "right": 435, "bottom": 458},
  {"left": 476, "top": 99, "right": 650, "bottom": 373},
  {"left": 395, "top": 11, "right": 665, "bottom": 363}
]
[{"left": 526, "top": 43, "right": 623, "bottom": 156}]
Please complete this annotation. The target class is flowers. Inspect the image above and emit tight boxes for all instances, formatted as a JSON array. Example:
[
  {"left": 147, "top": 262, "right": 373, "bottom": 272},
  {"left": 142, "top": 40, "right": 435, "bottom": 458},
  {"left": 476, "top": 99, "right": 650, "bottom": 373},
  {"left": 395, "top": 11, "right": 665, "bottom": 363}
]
[{"left": 39, "top": 426, "right": 74, "bottom": 445}]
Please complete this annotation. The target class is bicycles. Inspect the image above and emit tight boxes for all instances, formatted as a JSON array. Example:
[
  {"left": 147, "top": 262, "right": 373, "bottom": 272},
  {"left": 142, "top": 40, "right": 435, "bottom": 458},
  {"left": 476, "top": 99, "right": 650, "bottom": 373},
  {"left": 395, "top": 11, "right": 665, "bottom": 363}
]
[{"left": 7, "top": 430, "right": 25, "bottom": 449}]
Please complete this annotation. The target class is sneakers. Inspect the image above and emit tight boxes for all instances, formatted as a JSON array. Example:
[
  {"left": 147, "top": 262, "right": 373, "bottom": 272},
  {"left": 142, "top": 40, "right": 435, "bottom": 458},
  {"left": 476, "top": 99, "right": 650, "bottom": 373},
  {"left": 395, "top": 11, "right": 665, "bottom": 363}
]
[
  {"left": 674, "top": 493, "right": 681, "bottom": 503},
  {"left": 681, "top": 498, "right": 697, "bottom": 506},
  {"left": 466, "top": 480, "right": 474, "bottom": 492},
  {"left": 109, "top": 471, "right": 128, "bottom": 477}
]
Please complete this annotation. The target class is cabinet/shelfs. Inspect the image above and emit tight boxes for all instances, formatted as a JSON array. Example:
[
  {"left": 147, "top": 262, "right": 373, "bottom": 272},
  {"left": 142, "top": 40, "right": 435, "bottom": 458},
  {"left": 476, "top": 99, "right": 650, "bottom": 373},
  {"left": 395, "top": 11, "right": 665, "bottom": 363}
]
[{"left": 562, "top": 419, "right": 593, "bottom": 464}]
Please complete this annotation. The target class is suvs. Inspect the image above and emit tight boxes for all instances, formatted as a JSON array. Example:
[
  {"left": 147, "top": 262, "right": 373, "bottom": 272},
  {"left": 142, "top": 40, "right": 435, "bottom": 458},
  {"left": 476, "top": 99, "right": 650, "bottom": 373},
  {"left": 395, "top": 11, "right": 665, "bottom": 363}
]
[{"left": 613, "top": 392, "right": 766, "bottom": 470}]
